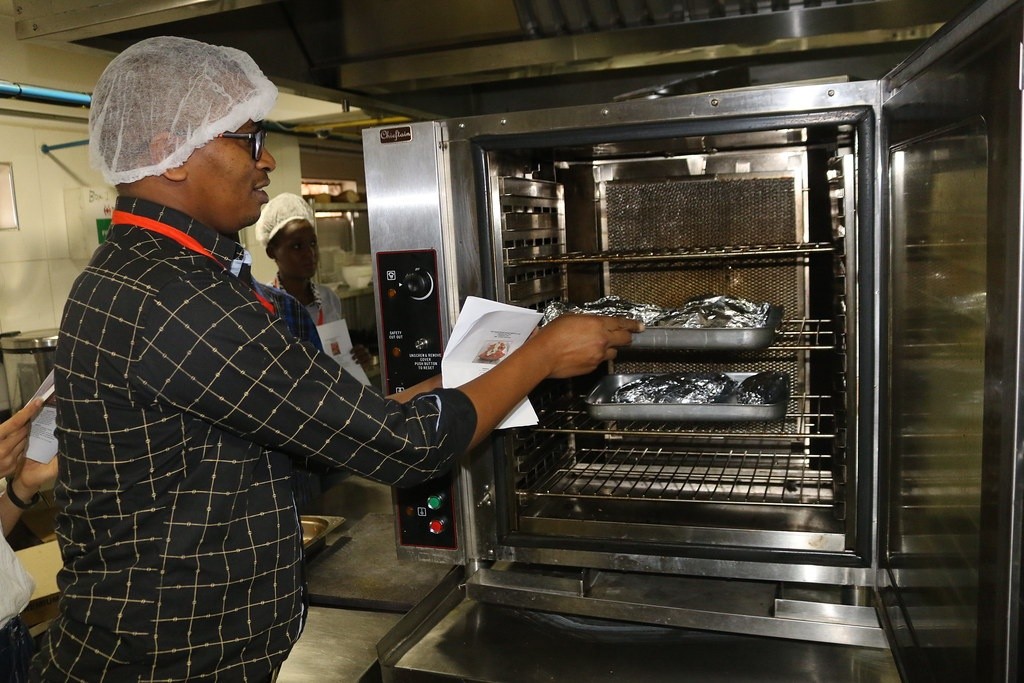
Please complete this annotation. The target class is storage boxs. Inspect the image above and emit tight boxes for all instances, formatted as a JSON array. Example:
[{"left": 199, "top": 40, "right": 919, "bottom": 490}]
[{"left": 7, "top": 491, "right": 64, "bottom": 651}]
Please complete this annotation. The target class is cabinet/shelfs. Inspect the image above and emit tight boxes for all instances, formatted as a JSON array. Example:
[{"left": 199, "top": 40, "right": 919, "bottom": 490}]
[
  {"left": 305, "top": 197, "right": 380, "bottom": 378},
  {"left": 513, "top": 243, "right": 844, "bottom": 511}
]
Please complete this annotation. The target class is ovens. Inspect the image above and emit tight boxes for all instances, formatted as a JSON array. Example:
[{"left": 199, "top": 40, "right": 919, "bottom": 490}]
[{"left": 359, "top": 77, "right": 884, "bottom": 587}]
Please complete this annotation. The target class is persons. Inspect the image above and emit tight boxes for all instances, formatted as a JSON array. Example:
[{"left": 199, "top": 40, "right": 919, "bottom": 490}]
[
  {"left": 27, "top": 36, "right": 644, "bottom": 683},
  {"left": 252, "top": 192, "right": 372, "bottom": 353}
]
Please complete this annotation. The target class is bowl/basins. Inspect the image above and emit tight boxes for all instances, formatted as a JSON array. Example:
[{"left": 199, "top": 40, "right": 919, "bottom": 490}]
[{"left": 341, "top": 266, "right": 373, "bottom": 290}]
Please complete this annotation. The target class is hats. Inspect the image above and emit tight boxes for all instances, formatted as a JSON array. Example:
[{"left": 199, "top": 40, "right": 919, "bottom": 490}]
[{"left": 255, "top": 191, "right": 316, "bottom": 251}]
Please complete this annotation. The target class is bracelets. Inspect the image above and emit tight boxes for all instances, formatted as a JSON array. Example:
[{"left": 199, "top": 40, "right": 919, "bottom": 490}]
[{"left": 7, "top": 478, "right": 39, "bottom": 509}]
